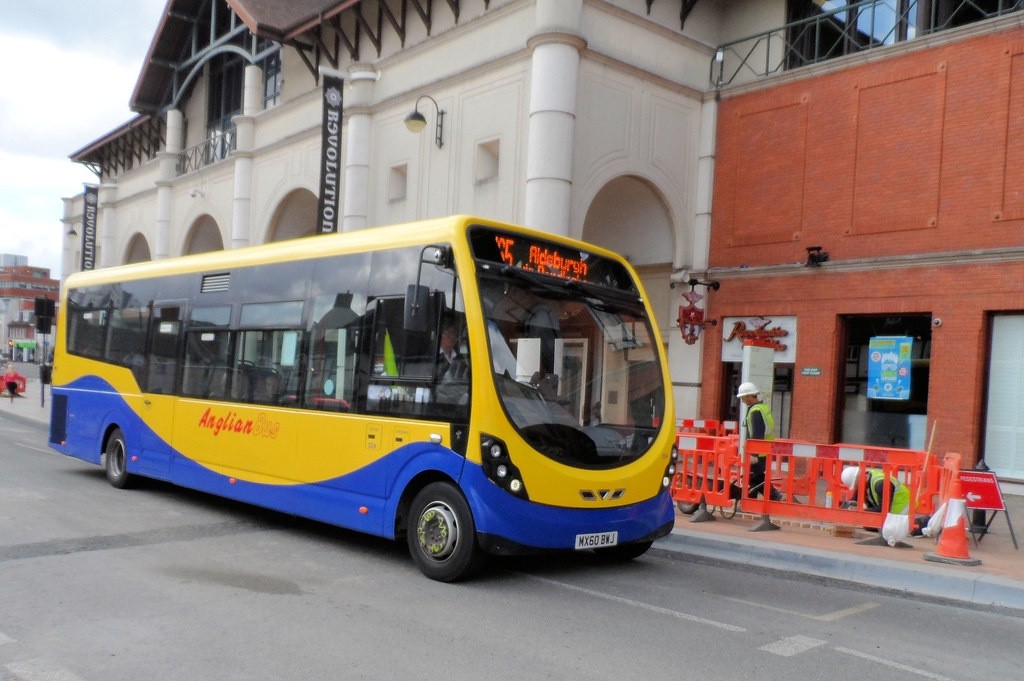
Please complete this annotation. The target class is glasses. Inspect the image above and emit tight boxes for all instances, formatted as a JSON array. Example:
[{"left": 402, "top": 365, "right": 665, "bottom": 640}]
[{"left": 443, "top": 330, "right": 457, "bottom": 336}]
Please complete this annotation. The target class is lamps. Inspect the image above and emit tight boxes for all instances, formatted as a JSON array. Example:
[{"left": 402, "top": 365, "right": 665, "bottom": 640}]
[
  {"left": 804, "top": 246, "right": 829, "bottom": 268},
  {"left": 66, "top": 219, "right": 82, "bottom": 240},
  {"left": 403, "top": 94, "right": 447, "bottom": 149}
]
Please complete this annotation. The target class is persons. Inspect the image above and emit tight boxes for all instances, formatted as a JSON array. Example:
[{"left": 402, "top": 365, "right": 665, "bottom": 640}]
[
  {"left": 431, "top": 323, "right": 465, "bottom": 381},
  {"left": 4, "top": 364, "right": 18, "bottom": 403},
  {"left": 841, "top": 465, "right": 920, "bottom": 533},
  {"left": 256, "top": 374, "right": 283, "bottom": 402},
  {"left": 736, "top": 381, "right": 803, "bottom": 504}
]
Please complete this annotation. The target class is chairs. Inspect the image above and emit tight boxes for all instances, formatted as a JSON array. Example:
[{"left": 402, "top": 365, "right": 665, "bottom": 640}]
[
  {"left": 398, "top": 321, "right": 431, "bottom": 363},
  {"left": 256, "top": 368, "right": 279, "bottom": 393},
  {"left": 237, "top": 360, "right": 258, "bottom": 390}
]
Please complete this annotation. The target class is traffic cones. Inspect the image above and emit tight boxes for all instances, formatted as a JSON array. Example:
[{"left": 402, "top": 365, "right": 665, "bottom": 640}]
[{"left": 922, "top": 477, "right": 983, "bottom": 567}]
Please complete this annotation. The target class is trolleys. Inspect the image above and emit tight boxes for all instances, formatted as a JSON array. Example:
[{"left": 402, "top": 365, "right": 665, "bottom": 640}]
[{"left": 675, "top": 470, "right": 783, "bottom": 519}]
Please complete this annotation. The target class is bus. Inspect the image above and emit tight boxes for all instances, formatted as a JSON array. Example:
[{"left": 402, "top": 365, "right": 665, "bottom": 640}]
[{"left": 46, "top": 215, "right": 676, "bottom": 584}]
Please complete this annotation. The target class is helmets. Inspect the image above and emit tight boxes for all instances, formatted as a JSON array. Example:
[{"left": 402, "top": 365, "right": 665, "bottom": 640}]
[
  {"left": 736, "top": 382, "right": 759, "bottom": 397},
  {"left": 841, "top": 467, "right": 859, "bottom": 490}
]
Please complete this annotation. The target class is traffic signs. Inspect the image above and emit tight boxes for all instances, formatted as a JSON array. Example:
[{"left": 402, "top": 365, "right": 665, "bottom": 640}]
[{"left": 957, "top": 470, "right": 1006, "bottom": 511}]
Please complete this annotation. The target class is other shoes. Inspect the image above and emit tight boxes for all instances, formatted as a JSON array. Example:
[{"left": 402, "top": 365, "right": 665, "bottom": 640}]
[
  {"left": 916, "top": 515, "right": 930, "bottom": 534},
  {"left": 775, "top": 494, "right": 782, "bottom": 500}
]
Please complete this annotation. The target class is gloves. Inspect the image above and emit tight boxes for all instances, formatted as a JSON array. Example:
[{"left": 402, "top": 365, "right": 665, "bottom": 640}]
[{"left": 751, "top": 455, "right": 759, "bottom": 465}]
